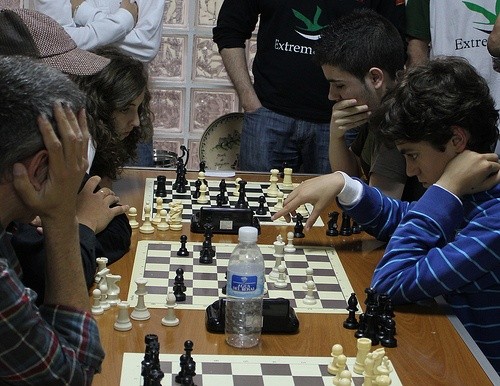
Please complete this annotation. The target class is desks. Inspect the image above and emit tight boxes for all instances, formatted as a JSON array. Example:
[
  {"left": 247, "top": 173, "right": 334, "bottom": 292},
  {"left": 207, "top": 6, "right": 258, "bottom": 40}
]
[{"left": 87, "top": 164, "right": 500, "bottom": 386}]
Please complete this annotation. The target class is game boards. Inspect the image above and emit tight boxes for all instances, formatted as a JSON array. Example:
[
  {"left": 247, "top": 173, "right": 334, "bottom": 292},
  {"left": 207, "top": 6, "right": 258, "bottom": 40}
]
[
  {"left": 126, "top": 238, "right": 364, "bottom": 316},
  {"left": 142, "top": 177, "right": 327, "bottom": 228},
  {"left": 119, "top": 352, "right": 404, "bottom": 386}
]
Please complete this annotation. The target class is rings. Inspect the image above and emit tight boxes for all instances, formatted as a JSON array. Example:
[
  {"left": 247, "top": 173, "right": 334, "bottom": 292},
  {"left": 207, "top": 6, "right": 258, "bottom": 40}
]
[{"left": 99, "top": 190, "right": 106, "bottom": 197}]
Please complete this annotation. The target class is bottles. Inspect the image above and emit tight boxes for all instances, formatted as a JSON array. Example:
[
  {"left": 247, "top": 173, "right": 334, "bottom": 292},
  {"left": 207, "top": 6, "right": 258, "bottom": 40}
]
[{"left": 225, "top": 225, "right": 266, "bottom": 349}]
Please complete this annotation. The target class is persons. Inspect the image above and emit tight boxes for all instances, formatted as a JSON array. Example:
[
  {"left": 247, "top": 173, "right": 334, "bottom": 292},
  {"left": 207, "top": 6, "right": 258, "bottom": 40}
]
[
  {"left": 316, "top": 11, "right": 423, "bottom": 202},
  {"left": 271, "top": 55, "right": 500, "bottom": 377},
  {"left": 0, "top": 0, "right": 165, "bottom": 306},
  {"left": 212, "top": 0, "right": 500, "bottom": 189},
  {"left": 0, "top": 54, "right": 105, "bottom": 386}
]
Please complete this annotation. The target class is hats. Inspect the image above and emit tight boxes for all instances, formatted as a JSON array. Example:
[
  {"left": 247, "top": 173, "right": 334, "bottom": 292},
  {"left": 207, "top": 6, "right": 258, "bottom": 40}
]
[{"left": 0, "top": 8, "right": 111, "bottom": 77}]
